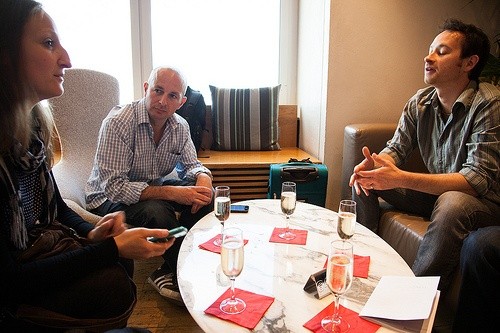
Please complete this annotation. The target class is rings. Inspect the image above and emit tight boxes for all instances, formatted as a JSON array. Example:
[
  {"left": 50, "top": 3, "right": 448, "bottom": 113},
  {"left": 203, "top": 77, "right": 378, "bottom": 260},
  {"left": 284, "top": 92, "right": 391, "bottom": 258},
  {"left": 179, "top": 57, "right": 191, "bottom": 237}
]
[{"left": 370, "top": 184, "right": 374, "bottom": 189}]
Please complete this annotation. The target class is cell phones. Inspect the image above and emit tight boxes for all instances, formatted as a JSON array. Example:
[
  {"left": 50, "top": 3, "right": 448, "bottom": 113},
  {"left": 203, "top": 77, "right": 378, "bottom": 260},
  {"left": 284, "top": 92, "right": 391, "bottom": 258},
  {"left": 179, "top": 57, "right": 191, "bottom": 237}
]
[
  {"left": 230, "top": 205, "right": 249, "bottom": 213},
  {"left": 148, "top": 226, "right": 187, "bottom": 244}
]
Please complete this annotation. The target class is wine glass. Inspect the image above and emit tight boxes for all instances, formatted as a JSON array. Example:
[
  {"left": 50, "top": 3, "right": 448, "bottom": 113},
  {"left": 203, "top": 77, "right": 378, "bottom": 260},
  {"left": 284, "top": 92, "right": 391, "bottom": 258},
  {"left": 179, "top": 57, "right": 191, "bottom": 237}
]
[
  {"left": 337, "top": 198, "right": 357, "bottom": 255},
  {"left": 219, "top": 226, "right": 247, "bottom": 316},
  {"left": 213, "top": 185, "right": 233, "bottom": 248},
  {"left": 321, "top": 239, "right": 354, "bottom": 333},
  {"left": 278, "top": 181, "right": 297, "bottom": 241}
]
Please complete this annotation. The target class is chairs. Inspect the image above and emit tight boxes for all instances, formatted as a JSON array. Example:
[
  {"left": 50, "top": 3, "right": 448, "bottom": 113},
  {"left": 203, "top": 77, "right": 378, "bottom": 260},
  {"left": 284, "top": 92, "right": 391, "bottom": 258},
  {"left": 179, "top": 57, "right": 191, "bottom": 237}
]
[{"left": 49, "top": 69, "right": 121, "bottom": 232}]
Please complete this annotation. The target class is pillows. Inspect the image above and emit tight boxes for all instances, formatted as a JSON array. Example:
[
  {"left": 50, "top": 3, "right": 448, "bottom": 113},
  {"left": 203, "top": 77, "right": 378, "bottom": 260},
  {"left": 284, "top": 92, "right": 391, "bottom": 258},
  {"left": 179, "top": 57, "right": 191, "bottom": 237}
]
[{"left": 210, "top": 84, "right": 282, "bottom": 151}]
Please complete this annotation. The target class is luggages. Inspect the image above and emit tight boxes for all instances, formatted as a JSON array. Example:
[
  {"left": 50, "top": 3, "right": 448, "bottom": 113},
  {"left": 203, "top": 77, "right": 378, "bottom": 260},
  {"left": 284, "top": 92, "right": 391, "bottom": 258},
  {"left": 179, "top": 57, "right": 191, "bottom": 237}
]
[{"left": 267, "top": 162, "right": 328, "bottom": 208}]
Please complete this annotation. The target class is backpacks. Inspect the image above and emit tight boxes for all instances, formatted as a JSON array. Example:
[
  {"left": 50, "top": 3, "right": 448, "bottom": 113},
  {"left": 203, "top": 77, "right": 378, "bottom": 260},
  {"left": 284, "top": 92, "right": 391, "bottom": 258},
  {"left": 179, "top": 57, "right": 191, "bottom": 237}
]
[{"left": 174, "top": 86, "right": 210, "bottom": 159}]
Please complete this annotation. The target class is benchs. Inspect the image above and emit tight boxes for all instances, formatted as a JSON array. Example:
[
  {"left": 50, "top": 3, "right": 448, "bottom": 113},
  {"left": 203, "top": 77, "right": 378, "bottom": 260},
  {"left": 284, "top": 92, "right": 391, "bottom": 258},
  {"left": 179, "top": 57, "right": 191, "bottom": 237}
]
[{"left": 178, "top": 105, "right": 322, "bottom": 203}]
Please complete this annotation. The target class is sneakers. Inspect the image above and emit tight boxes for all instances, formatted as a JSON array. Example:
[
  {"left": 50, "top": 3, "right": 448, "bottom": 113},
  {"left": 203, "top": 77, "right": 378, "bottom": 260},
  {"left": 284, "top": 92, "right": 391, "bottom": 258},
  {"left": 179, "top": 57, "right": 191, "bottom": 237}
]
[{"left": 147, "top": 266, "right": 185, "bottom": 307}]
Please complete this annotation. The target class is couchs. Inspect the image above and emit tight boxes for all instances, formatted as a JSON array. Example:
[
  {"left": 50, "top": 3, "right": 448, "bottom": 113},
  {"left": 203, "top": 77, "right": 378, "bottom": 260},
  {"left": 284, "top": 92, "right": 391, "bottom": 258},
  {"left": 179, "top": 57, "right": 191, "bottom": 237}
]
[{"left": 341, "top": 122, "right": 465, "bottom": 288}]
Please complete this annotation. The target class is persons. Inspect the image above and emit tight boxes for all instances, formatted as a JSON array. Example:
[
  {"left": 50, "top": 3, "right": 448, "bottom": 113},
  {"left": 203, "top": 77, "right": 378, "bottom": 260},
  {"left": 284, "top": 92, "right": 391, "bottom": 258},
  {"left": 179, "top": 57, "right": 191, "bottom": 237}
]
[
  {"left": 0, "top": 0, "right": 174, "bottom": 333},
  {"left": 452, "top": 225, "right": 500, "bottom": 333},
  {"left": 85, "top": 66, "right": 215, "bottom": 302},
  {"left": 348, "top": 17, "right": 500, "bottom": 303}
]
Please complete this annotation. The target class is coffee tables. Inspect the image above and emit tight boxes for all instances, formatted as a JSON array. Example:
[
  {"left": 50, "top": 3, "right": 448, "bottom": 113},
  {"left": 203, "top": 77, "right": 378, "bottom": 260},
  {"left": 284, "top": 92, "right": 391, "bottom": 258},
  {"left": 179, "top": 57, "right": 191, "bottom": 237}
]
[{"left": 176, "top": 198, "right": 417, "bottom": 333}]
